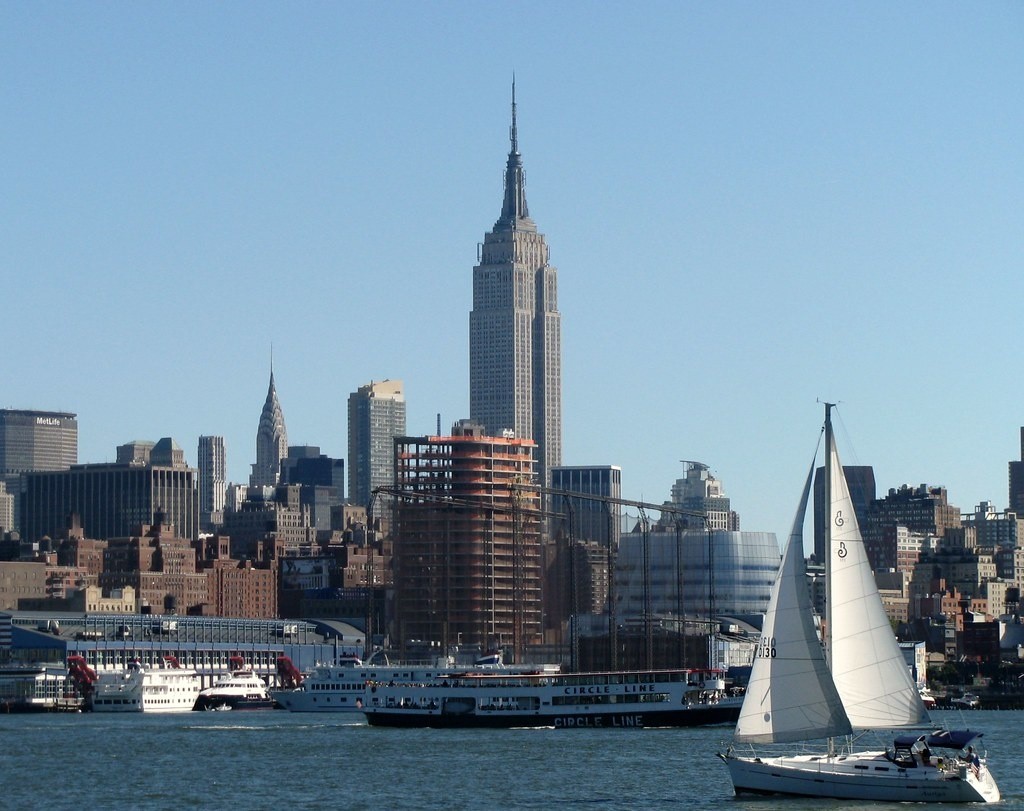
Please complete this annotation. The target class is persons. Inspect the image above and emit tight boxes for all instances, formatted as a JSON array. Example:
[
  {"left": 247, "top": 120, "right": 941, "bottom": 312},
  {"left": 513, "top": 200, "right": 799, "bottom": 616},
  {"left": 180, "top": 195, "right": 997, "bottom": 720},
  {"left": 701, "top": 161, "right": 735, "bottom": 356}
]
[
  {"left": 937, "top": 759, "right": 943, "bottom": 769},
  {"left": 365, "top": 679, "right": 557, "bottom": 688},
  {"left": 958, "top": 746, "right": 974, "bottom": 763},
  {"left": 387, "top": 702, "right": 438, "bottom": 710},
  {"left": 478, "top": 704, "right": 527, "bottom": 711}
]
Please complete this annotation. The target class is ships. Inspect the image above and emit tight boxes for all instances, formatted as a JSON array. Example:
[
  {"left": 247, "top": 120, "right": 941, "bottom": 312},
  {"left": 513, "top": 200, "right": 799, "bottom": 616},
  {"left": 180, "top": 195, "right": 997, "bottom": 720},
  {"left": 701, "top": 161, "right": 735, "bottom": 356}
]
[
  {"left": 265, "top": 646, "right": 562, "bottom": 712},
  {"left": 360, "top": 667, "right": 744, "bottom": 729}
]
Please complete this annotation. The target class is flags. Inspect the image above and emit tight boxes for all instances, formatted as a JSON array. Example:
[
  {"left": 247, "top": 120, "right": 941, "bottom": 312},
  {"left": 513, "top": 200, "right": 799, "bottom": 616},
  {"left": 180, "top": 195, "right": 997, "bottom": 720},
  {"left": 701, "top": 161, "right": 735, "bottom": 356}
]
[{"left": 971, "top": 754, "right": 980, "bottom": 781}]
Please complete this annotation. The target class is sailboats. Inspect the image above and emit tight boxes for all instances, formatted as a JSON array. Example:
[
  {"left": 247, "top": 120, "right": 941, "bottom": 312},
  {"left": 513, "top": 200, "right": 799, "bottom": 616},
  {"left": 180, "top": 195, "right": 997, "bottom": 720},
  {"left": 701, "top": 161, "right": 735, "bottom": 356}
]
[{"left": 717, "top": 396, "right": 1000, "bottom": 804}]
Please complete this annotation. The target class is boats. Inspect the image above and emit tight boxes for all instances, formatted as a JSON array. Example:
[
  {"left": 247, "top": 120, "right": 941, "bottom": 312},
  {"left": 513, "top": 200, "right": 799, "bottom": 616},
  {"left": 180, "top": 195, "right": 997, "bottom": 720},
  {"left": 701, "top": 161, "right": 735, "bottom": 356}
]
[
  {"left": 90, "top": 668, "right": 202, "bottom": 712},
  {"left": 192, "top": 670, "right": 286, "bottom": 710},
  {"left": 950, "top": 693, "right": 983, "bottom": 711},
  {"left": 917, "top": 690, "right": 934, "bottom": 711},
  {"left": 212, "top": 703, "right": 232, "bottom": 711}
]
